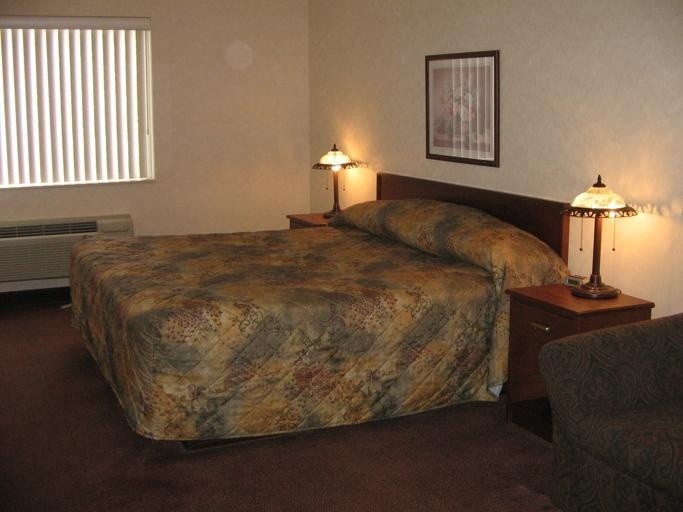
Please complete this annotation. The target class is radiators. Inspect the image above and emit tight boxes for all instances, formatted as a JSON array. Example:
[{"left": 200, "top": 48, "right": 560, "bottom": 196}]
[{"left": 0, "top": 212, "right": 134, "bottom": 294}]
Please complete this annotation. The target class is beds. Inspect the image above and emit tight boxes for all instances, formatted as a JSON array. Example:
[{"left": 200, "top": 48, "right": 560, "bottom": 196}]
[{"left": 69, "top": 170, "right": 570, "bottom": 441}]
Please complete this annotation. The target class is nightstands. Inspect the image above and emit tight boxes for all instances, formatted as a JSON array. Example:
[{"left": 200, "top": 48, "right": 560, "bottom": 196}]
[
  {"left": 505, "top": 282, "right": 655, "bottom": 446},
  {"left": 286, "top": 214, "right": 343, "bottom": 229}
]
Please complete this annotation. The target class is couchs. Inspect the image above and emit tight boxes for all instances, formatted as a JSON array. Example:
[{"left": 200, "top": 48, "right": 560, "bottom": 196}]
[{"left": 536, "top": 313, "right": 683, "bottom": 511}]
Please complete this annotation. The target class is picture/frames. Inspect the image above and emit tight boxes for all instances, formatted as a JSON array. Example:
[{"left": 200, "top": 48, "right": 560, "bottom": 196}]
[{"left": 425, "top": 48, "right": 502, "bottom": 168}]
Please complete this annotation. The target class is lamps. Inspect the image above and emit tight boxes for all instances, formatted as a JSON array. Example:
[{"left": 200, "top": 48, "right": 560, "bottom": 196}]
[
  {"left": 311, "top": 143, "right": 360, "bottom": 219},
  {"left": 561, "top": 174, "right": 638, "bottom": 301}
]
